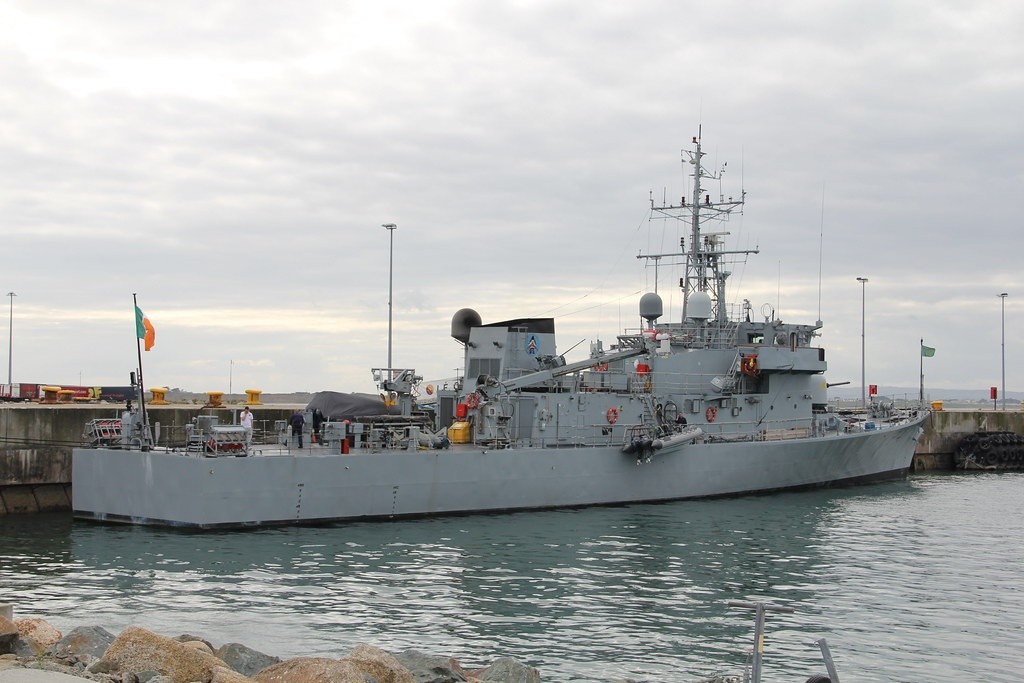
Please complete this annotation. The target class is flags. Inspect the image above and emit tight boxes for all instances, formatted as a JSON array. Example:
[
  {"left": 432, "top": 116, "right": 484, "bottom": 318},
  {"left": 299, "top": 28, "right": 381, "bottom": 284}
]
[
  {"left": 921, "top": 345, "right": 935, "bottom": 357},
  {"left": 143, "top": 318, "right": 156, "bottom": 351},
  {"left": 135, "top": 306, "right": 146, "bottom": 339}
]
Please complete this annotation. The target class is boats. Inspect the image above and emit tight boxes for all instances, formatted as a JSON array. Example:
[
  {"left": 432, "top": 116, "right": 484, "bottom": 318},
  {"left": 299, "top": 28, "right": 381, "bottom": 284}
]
[{"left": 622, "top": 424, "right": 702, "bottom": 455}]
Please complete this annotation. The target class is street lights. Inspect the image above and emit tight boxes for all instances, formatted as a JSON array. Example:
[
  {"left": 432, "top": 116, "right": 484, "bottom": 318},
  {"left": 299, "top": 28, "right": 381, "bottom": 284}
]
[
  {"left": 855, "top": 277, "right": 868, "bottom": 408},
  {"left": 382, "top": 223, "right": 398, "bottom": 381},
  {"left": 998, "top": 291, "right": 1009, "bottom": 411}
]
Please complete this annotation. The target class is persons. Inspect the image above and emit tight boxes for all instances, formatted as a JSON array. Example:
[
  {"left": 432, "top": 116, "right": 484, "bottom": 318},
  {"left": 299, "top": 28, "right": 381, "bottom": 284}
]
[
  {"left": 281, "top": 409, "right": 304, "bottom": 448},
  {"left": 240, "top": 406, "right": 254, "bottom": 450},
  {"left": 676, "top": 411, "right": 687, "bottom": 428},
  {"left": 308, "top": 408, "right": 324, "bottom": 446}
]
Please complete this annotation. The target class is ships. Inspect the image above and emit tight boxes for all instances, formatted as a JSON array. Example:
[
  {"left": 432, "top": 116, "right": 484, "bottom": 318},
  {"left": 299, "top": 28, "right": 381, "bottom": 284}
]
[{"left": 70, "top": 125, "right": 932, "bottom": 531}]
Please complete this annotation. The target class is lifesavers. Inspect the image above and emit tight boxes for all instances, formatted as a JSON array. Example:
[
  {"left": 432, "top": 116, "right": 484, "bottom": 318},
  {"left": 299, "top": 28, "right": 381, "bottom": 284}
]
[
  {"left": 744, "top": 360, "right": 757, "bottom": 374},
  {"left": 706, "top": 407, "right": 717, "bottom": 422},
  {"left": 607, "top": 407, "right": 618, "bottom": 424},
  {"left": 466, "top": 392, "right": 480, "bottom": 409},
  {"left": 594, "top": 362, "right": 608, "bottom": 372}
]
